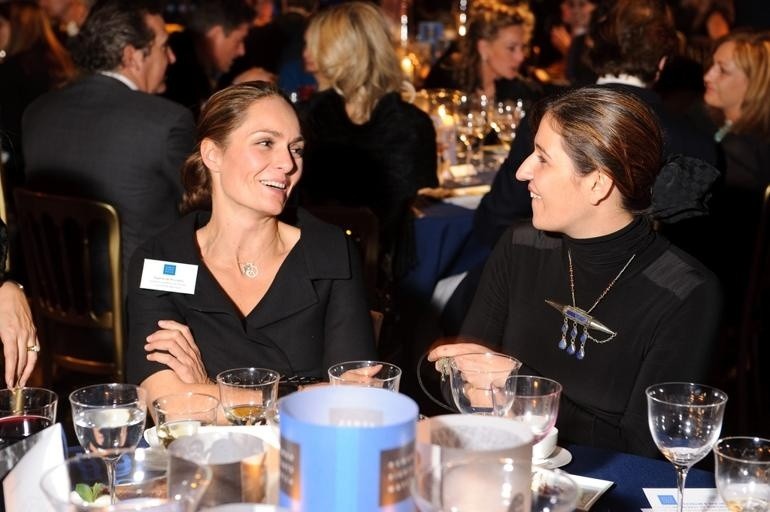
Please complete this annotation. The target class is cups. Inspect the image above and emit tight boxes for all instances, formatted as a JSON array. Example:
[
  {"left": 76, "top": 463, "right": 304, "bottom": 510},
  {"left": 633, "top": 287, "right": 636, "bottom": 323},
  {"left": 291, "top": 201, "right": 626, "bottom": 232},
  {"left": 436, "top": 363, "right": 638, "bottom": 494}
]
[{"left": 711, "top": 435, "right": 770, "bottom": 512}]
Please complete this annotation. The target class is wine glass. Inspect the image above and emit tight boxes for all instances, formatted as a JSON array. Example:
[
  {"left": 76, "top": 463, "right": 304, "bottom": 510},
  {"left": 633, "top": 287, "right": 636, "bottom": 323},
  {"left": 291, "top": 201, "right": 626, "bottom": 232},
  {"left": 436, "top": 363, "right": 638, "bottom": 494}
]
[
  {"left": 0, "top": 349, "right": 583, "bottom": 512},
  {"left": 425, "top": 88, "right": 526, "bottom": 175},
  {"left": 643, "top": 381, "right": 729, "bottom": 511}
]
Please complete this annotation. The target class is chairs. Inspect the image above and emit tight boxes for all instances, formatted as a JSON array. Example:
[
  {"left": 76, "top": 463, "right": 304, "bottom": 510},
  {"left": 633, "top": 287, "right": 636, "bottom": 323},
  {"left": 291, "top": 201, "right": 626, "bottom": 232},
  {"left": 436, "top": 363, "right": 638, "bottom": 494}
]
[
  {"left": 309, "top": 204, "right": 382, "bottom": 304},
  {"left": 10, "top": 184, "right": 132, "bottom": 447}
]
